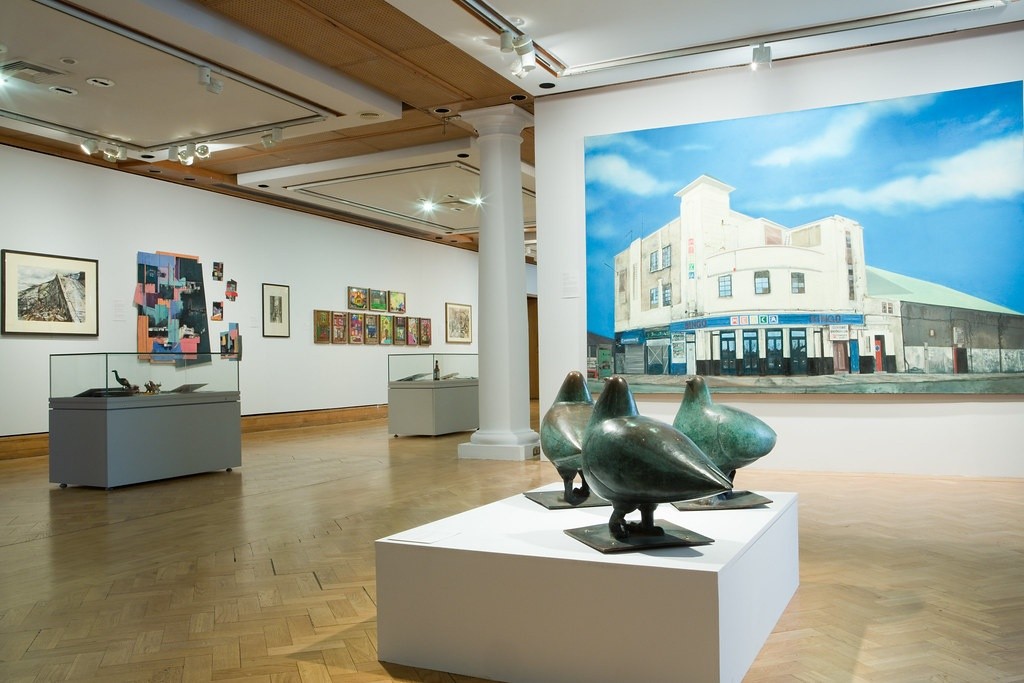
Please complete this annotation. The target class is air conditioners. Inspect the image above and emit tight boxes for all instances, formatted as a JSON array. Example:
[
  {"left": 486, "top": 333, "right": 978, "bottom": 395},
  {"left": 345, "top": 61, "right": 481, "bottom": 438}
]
[{"left": 272, "top": 128, "right": 283, "bottom": 143}]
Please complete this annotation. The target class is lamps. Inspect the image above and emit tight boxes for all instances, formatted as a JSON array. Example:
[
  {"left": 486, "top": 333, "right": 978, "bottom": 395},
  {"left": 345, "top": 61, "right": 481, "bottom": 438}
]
[
  {"left": 187, "top": 143, "right": 196, "bottom": 157},
  {"left": 103, "top": 148, "right": 119, "bottom": 159},
  {"left": 512, "top": 34, "right": 534, "bottom": 56},
  {"left": 208, "top": 77, "right": 224, "bottom": 95},
  {"left": 168, "top": 145, "right": 180, "bottom": 162},
  {"left": 196, "top": 145, "right": 211, "bottom": 158},
  {"left": 500, "top": 32, "right": 513, "bottom": 53},
  {"left": 510, "top": 59, "right": 530, "bottom": 80},
  {"left": 81, "top": 137, "right": 98, "bottom": 156},
  {"left": 118, "top": 146, "right": 128, "bottom": 161},
  {"left": 180, "top": 149, "right": 195, "bottom": 166},
  {"left": 521, "top": 50, "right": 536, "bottom": 70},
  {"left": 198, "top": 67, "right": 210, "bottom": 85},
  {"left": 752, "top": 43, "right": 773, "bottom": 68},
  {"left": 259, "top": 130, "right": 277, "bottom": 148}
]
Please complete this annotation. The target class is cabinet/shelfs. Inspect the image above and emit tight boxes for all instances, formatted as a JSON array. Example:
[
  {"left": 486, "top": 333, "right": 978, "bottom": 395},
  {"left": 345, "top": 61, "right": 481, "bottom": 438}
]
[
  {"left": 386, "top": 386, "right": 479, "bottom": 437},
  {"left": 49, "top": 400, "right": 242, "bottom": 490}
]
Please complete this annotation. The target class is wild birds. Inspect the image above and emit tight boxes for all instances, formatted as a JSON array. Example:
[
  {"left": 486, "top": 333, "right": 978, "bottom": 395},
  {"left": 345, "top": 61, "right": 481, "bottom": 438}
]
[
  {"left": 582, "top": 377, "right": 733, "bottom": 540},
  {"left": 671, "top": 374, "right": 775, "bottom": 497},
  {"left": 112, "top": 369, "right": 131, "bottom": 388},
  {"left": 540, "top": 371, "right": 595, "bottom": 506}
]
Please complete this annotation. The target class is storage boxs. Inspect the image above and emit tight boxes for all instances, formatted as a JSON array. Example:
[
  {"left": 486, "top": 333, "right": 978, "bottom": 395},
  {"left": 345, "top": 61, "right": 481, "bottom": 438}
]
[
  {"left": 387, "top": 353, "right": 479, "bottom": 384},
  {"left": 49, "top": 351, "right": 240, "bottom": 398}
]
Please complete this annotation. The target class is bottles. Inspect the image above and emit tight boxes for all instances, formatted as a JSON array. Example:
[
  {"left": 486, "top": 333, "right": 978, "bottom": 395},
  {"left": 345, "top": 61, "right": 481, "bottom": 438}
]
[{"left": 434, "top": 360, "right": 440, "bottom": 380}]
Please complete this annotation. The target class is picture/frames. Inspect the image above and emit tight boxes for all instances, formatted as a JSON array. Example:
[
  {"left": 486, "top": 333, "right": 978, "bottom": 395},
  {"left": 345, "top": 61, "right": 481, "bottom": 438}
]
[
  {"left": 349, "top": 312, "right": 365, "bottom": 344},
  {"left": 389, "top": 290, "right": 407, "bottom": 314},
  {"left": 408, "top": 317, "right": 420, "bottom": 346},
  {"left": 332, "top": 311, "right": 349, "bottom": 344},
  {"left": 1, "top": 249, "right": 99, "bottom": 337},
  {"left": 420, "top": 318, "right": 432, "bottom": 347},
  {"left": 313, "top": 308, "right": 332, "bottom": 344},
  {"left": 379, "top": 315, "right": 394, "bottom": 345},
  {"left": 364, "top": 313, "right": 380, "bottom": 346},
  {"left": 261, "top": 282, "right": 291, "bottom": 338},
  {"left": 445, "top": 302, "right": 473, "bottom": 344},
  {"left": 393, "top": 317, "right": 408, "bottom": 346},
  {"left": 347, "top": 286, "right": 370, "bottom": 310},
  {"left": 370, "top": 289, "right": 388, "bottom": 312}
]
